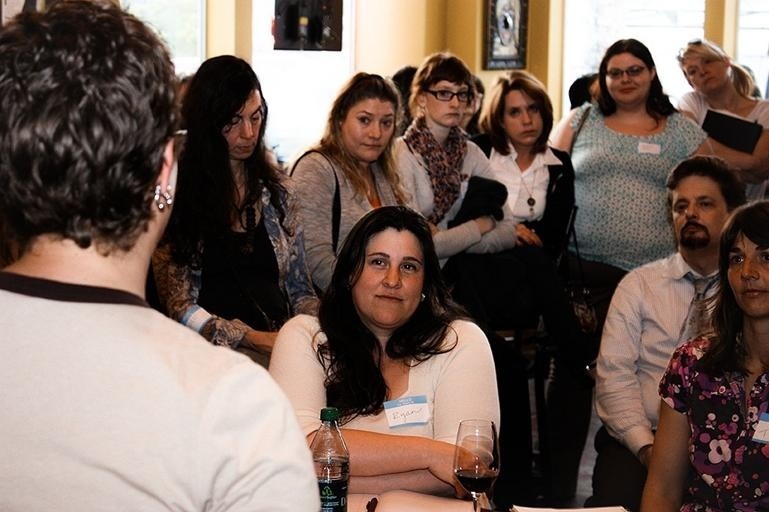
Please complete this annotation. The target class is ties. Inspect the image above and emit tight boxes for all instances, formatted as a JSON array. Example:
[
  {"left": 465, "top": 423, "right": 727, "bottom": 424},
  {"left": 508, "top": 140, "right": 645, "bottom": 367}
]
[{"left": 676, "top": 271, "right": 721, "bottom": 351}]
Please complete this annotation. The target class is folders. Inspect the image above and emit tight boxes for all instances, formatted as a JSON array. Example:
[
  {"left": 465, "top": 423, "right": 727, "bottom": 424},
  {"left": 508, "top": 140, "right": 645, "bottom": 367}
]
[{"left": 701, "top": 107, "right": 763, "bottom": 156}]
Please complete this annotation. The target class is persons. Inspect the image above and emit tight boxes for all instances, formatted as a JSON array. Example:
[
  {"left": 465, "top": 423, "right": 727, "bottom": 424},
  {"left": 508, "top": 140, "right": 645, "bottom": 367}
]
[
  {"left": 468, "top": 39, "right": 769, "bottom": 512},
  {"left": 1, "top": 0, "right": 516, "bottom": 512}
]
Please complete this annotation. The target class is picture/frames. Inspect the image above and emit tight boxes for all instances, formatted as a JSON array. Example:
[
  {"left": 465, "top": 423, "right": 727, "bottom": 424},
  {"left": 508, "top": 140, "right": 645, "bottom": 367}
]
[
  {"left": 271, "top": 0, "right": 342, "bottom": 50},
  {"left": 484, "top": 0, "right": 528, "bottom": 71}
]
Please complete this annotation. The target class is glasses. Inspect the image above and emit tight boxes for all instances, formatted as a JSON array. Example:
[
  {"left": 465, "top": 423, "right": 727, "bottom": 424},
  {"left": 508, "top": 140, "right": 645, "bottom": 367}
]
[
  {"left": 422, "top": 89, "right": 476, "bottom": 103},
  {"left": 606, "top": 65, "right": 647, "bottom": 80}
]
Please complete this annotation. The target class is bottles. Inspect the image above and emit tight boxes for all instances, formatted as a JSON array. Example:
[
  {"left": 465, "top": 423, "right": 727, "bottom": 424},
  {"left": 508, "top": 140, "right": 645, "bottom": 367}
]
[{"left": 307, "top": 407, "right": 352, "bottom": 512}]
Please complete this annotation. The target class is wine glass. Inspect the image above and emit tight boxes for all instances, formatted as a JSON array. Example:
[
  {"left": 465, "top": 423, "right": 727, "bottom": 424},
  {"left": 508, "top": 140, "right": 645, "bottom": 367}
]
[{"left": 452, "top": 418, "right": 502, "bottom": 511}]
